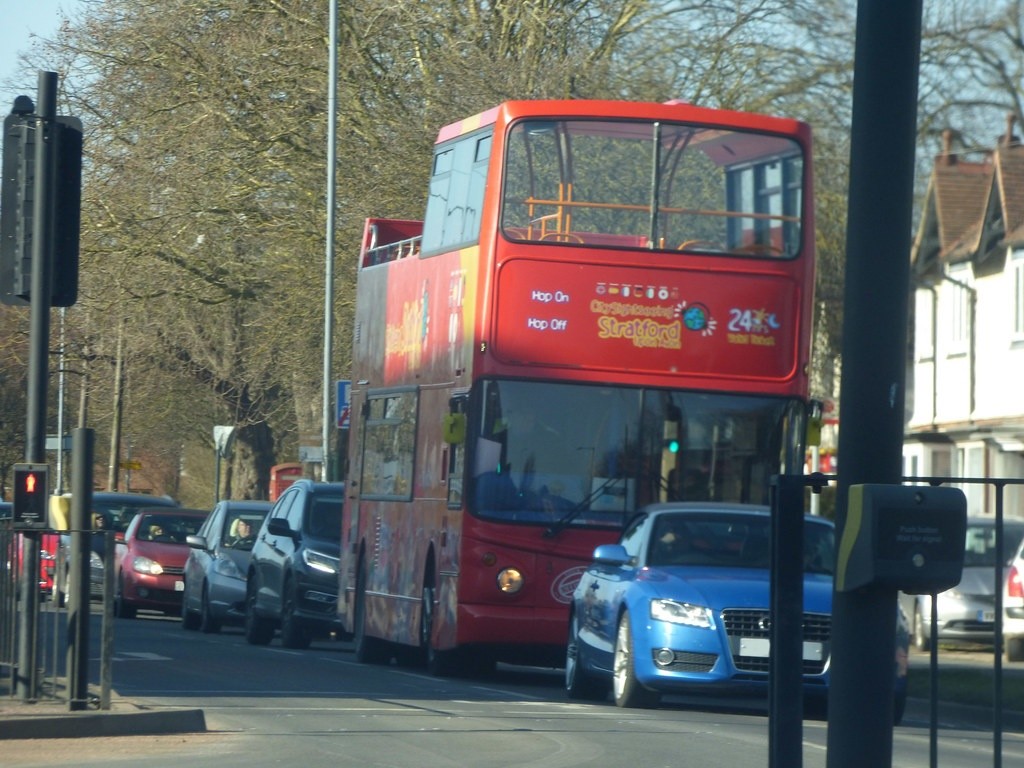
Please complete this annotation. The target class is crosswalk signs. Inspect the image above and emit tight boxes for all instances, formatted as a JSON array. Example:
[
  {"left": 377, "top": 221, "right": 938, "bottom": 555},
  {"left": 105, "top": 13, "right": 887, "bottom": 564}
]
[{"left": 13, "top": 463, "right": 50, "bottom": 531}]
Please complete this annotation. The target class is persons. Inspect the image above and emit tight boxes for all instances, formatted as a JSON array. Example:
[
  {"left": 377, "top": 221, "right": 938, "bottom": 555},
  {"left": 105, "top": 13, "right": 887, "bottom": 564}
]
[
  {"left": 146, "top": 524, "right": 164, "bottom": 540},
  {"left": 91, "top": 512, "right": 106, "bottom": 530},
  {"left": 225, "top": 519, "right": 257, "bottom": 549}
]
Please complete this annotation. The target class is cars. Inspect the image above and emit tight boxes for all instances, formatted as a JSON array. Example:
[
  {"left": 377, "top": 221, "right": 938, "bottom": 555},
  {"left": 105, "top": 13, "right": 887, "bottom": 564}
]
[
  {"left": 565, "top": 501, "right": 912, "bottom": 731},
  {"left": 181, "top": 498, "right": 279, "bottom": 633},
  {"left": 896, "top": 508, "right": 1023, "bottom": 654},
  {"left": 8, "top": 513, "right": 63, "bottom": 593},
  {"left": 1003, "top": 538, "right": 1024, "bottom": 661},
  {"left": 113, "top": 511, "right": 210, "bottom": 618}
]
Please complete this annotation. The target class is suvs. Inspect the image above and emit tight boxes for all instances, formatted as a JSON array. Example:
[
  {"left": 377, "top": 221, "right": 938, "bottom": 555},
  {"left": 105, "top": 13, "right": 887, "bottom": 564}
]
[
  {"left": 59, "top": 490, "right": 180, "bottom": 611},
  {"left": 243, "top": 476, "right": 347, "bottom": 653}
]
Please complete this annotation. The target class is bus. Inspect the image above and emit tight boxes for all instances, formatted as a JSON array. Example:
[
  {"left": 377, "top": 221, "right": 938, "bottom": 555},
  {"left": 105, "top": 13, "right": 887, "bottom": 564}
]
[{"left": 336, "top": 99, "right": 820, "bottom": 680}]
